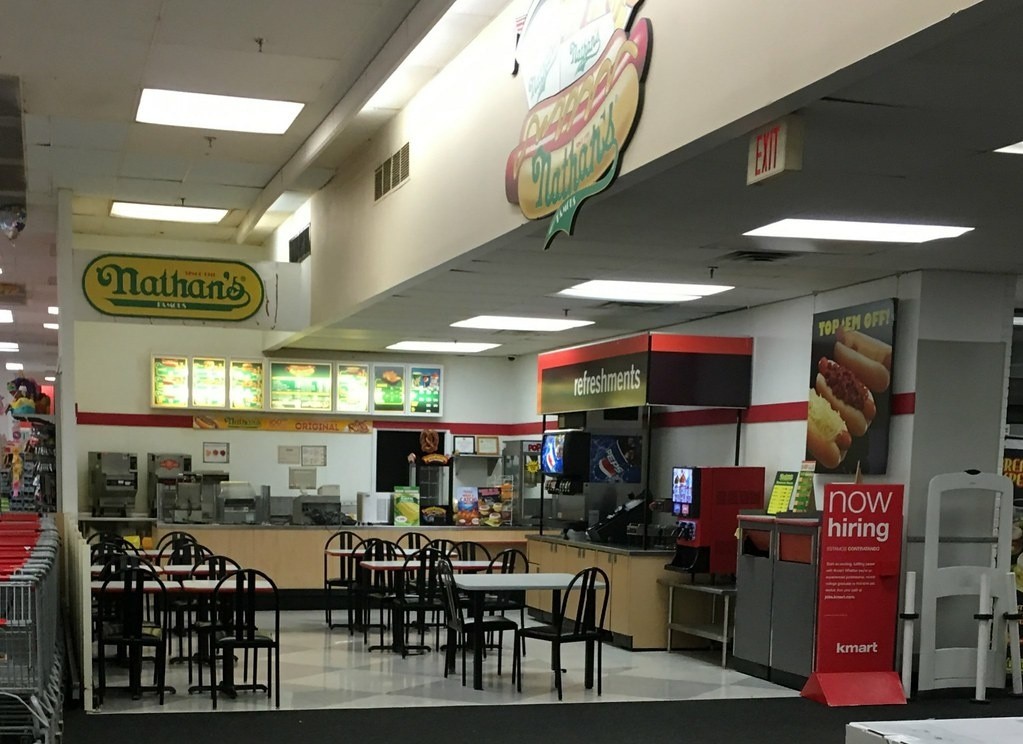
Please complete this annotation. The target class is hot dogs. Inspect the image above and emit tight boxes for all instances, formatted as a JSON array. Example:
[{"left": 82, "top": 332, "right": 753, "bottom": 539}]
[
  {"left": 832, "top": 326, "right": 891, "bottom": 394},
  {"left": 816, "top": 357, "right": 876, "bottom": 437},
  {"left": 160, "top": 358, "right": 179, "bottom": 399},
  {"left": 806, "top": 387, "right": 852, "bottom": 469},
  {"left": 287, "top": 365, "right": 315, "bottom": 377},
  {"left": 195, "top": 415, "right": 217, "bottom": 429}
]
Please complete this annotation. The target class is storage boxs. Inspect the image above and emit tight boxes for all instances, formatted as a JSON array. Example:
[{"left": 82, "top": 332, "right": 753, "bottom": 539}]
[{"left": 846, "top": 717, "right": 1023, "bottom": 744}]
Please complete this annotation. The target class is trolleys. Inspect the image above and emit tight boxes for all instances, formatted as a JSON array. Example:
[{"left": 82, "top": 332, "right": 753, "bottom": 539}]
[{"left": 0, "top": 512, "right": 68, "bottom": 744}]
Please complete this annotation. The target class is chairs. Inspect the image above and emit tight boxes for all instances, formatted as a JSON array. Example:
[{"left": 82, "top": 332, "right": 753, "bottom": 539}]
[
  {"left": 324, "top": 531, "right": 610, "bottom": 702},
  {"left": 86, "top": 531, "right": 280, "bottom": 711}
]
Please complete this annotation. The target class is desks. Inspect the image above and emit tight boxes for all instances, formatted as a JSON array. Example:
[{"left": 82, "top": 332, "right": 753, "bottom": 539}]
[
  {"left": 360, "top": 561, "right": 516, "bottom": 652},
  {"left": 92, "top": 549, "right": 209, "bottom": 637},
  {"left": 436, "top": 573, "right": 607, "bottom": 691},
  {"left": 656, "top": 579, "right": 737, "bottom": 670},
  {"left": 324, "top": 550, "right": 459, "bottom": 632},
  {"left": 91, "top": 564, "right": 237, "bottom": 665},
  {"left": 92, "top": 580, "right": 275, "bottom": 700}
]
